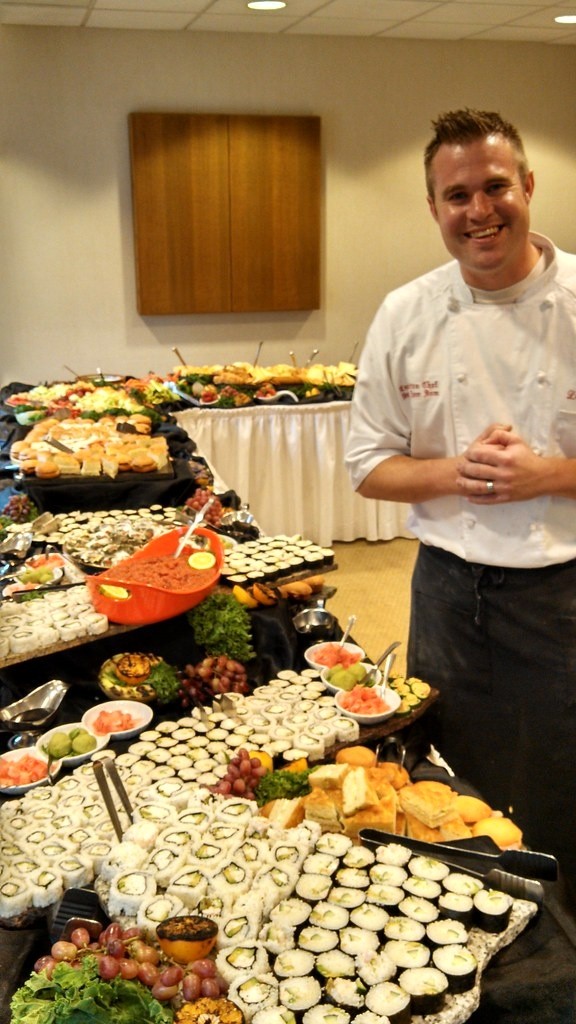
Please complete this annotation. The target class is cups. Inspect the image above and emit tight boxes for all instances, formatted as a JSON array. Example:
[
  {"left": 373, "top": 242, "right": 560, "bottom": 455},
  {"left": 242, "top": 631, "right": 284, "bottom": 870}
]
[{"left": 220, "top": 503, "right": 254, "bottom": 529}]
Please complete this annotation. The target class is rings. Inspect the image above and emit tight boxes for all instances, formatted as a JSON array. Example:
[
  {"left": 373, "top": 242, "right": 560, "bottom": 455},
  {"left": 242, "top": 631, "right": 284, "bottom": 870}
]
[{"left": 487, "top": 480, "right": 494, "bottom": 494}]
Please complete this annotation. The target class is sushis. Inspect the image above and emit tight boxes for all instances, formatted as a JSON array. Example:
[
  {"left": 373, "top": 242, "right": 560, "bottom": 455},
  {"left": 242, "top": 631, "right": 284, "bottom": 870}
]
[
  {"left": 209, "top": 532, "right": 336, "bottom": 587},
  {"left": 4, "top": 503, "right": 184, "bottom": 552},
  {"left": 0, "top": 668, "right": 515, "bottom": 1024},
  {"left": 0, "top": 585, "right": 109, "bottom": 661}
]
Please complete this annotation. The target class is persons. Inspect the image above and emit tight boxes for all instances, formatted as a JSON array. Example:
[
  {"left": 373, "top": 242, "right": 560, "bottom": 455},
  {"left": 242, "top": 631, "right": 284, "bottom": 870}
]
[{"left": 345, "top": 105, "right": 576, "bottom": 920}]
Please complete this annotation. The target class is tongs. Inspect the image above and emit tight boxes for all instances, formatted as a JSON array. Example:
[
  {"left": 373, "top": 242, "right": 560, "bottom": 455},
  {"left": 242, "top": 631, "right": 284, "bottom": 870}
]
[
  {"left": 117, "top": 422, "right": 150, "bottom": 436},
  {"left": 358, "top": 826, "right": 558, "bottom": 905},
  {"left": 93, "top": 756, "right": 136, "bottom": 844},
  {"left": 322, "top": 370, "right": 343, "bottom": 398},
  {"left": 175, "top": 668, "right": 237, "bottom": 729},
  {"left": 43, "top": 435, "right": 75, "bottom": 455},
  {"left": 12, "top": 582, "right": 85, "bottom": 603},
  {"left": 45, "top": 543, "right": 67, "bottom": 566}
]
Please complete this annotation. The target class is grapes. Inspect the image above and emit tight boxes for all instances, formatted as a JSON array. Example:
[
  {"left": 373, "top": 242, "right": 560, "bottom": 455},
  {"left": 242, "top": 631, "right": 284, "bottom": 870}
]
[
  {"left": 34, "top": 923, "right": 160, "bottom": 986},
  {"left": 151, "top": 956, "right": 228, "bottom": 1007},
  {"left": 198, "top": 748, "right": 266, "bottom": 801},
  {"left": 185, "top": 488, "right": 225, "bottom": 528},
  {"left": 173, "top": 651, "right": 250, "bottom": 711},
  {"left": 2, "top": 494, "right": 32, "bottom": 523}
]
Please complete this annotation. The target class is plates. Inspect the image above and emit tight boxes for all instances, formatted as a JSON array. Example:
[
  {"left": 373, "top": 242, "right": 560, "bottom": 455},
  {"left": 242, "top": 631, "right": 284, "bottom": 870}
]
[
  {"left": 0, "top": 361, "right": 576, "bottom": 1024},
  {"left": 85, "top": 525, "right": 224, "bottom": 627},
  {"left": 305, "top": 640, "right": 401, "bottom": 723},
  {"left": 0, "top": 700, "right": 154, "bottom": 794}
]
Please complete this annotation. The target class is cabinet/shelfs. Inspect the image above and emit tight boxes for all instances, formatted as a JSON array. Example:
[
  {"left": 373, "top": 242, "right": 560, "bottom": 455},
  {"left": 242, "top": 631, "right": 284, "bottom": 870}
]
[{"left": 127, "top": 112, "right": 323, "bottom": 316}]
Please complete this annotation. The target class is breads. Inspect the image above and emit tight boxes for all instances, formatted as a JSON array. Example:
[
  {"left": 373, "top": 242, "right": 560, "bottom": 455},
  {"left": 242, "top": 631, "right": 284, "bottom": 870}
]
[{"left": 9, "top": 411, "right": 167, "bottom": 478}]
